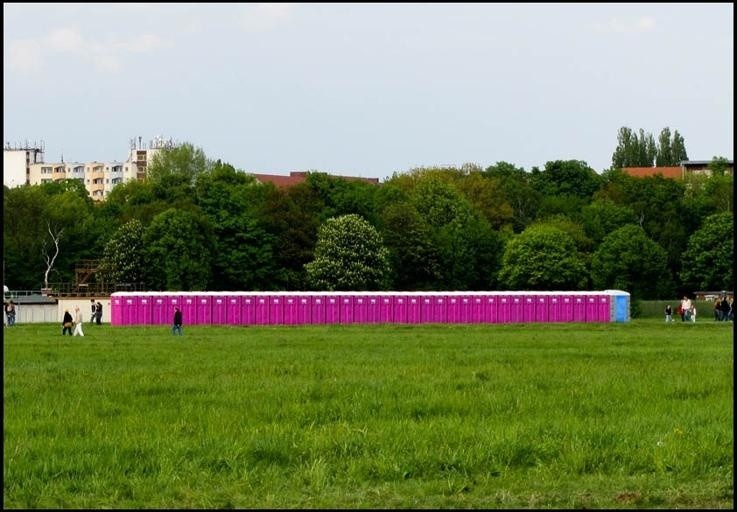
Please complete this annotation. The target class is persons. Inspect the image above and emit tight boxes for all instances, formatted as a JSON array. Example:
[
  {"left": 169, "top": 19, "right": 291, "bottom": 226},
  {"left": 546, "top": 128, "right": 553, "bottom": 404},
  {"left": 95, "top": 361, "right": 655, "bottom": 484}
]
[
  {"left": 665, "top": 296, "right": 696, "bottom": 323},
  {"left": 5, "top": 300, "right": 15, "bottom": 325},
  {"left": 90, "top": 299, "right": 102, "bottom": 326},
  {"left": 63, "top": 306, "right": 84, "bottom": 336},
  {"left": 714, "top": 296, "right": 734, "bottom": 321},
  {"left": 172, "top": 306, "right": 182, "bottom": 335}
]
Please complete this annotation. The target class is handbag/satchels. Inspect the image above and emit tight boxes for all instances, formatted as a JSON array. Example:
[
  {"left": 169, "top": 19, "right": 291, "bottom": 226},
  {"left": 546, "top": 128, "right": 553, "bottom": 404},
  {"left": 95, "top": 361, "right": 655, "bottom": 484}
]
[{"left": 64, "top": 323, "right": 71, "bottom": 327}]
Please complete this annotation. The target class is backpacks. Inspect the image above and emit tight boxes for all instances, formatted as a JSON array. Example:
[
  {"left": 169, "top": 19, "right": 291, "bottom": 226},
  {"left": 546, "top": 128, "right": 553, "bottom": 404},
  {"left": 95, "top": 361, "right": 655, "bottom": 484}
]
[{"left": 7, "top": 305, "right": 12, "bottom": 312}]
